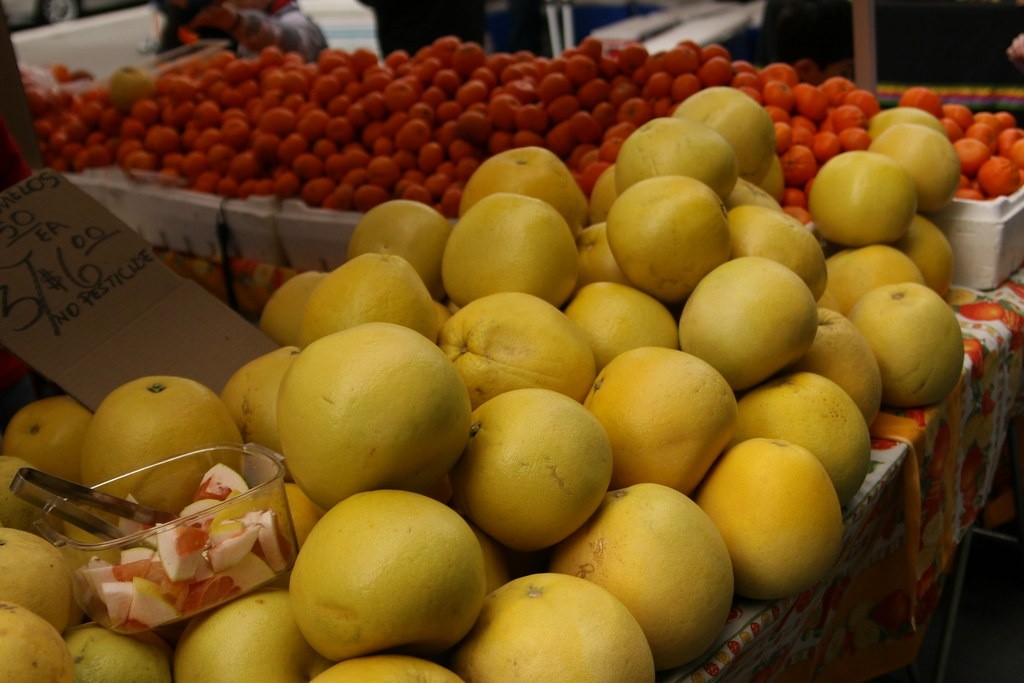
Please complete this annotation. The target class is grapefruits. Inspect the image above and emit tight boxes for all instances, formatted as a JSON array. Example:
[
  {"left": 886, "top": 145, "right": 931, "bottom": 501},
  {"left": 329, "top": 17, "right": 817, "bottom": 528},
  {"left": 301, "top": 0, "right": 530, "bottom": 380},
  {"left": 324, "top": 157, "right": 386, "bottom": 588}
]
[{"left": 0, "top": 87, "right": 968, "bottom": 683}]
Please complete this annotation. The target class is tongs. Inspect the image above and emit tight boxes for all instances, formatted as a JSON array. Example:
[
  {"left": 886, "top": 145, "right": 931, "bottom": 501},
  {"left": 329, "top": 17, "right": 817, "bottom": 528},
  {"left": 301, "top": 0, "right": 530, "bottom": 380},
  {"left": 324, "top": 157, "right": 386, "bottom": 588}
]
[{"left": 10, "top": 468, "right": 181, "bottom": 550}]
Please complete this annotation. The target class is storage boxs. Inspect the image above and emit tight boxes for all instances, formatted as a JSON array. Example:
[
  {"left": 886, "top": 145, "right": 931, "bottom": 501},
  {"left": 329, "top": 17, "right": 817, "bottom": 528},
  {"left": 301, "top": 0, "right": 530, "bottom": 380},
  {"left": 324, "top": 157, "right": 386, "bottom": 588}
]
[
  {"left": 8, "top": 2, "right": 166, "bottom": 87},
  {"left": 944, "top": 183, "right": 1024, "bottom": 291}
]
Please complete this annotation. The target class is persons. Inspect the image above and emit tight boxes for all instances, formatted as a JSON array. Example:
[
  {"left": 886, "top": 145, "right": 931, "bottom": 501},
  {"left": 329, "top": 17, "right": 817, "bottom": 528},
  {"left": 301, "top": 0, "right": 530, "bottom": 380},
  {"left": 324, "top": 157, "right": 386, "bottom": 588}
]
[
  {"left": 360, "top": 0, "right": 1024, "bottom": 93},
  {"left": 147, "top": 1, "right": 328, "bottom": 55}
]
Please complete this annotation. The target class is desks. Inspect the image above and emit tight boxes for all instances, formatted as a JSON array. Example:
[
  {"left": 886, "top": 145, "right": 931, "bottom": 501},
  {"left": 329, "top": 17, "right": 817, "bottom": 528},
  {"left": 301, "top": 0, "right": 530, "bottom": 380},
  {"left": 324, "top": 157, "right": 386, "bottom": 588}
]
[{"left": 62, "top": 163, "right": 1024, "bottom": 683}]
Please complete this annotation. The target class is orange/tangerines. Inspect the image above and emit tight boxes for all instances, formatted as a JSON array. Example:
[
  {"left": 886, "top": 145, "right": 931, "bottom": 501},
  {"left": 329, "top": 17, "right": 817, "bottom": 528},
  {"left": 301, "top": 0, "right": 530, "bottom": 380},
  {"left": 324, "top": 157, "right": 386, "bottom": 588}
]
[{"left": 15, "top": 37, "right": 1024, "bottom": 225}]
